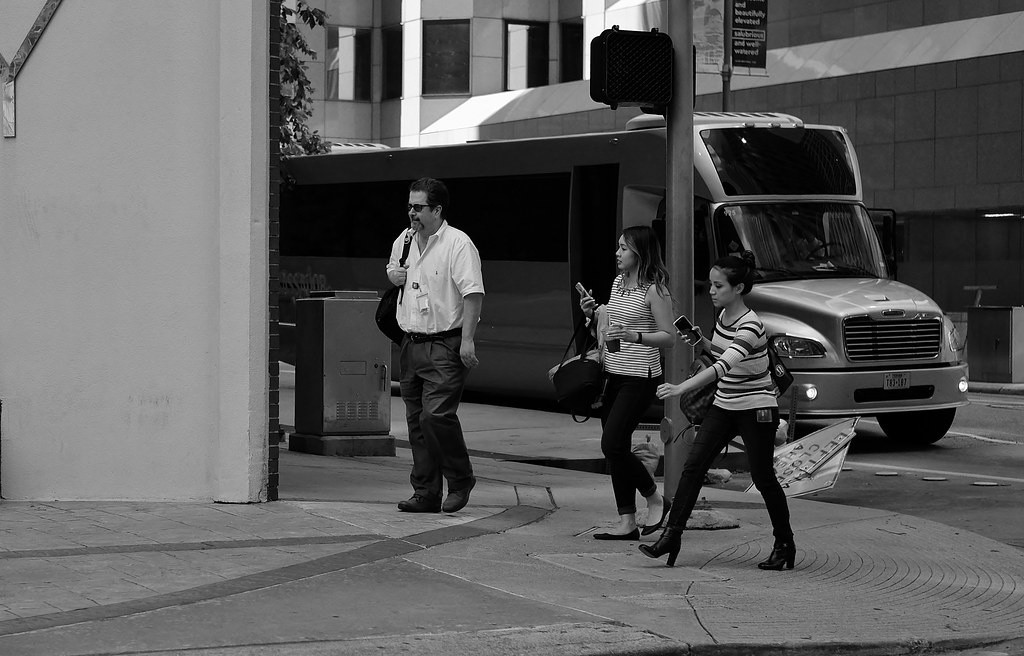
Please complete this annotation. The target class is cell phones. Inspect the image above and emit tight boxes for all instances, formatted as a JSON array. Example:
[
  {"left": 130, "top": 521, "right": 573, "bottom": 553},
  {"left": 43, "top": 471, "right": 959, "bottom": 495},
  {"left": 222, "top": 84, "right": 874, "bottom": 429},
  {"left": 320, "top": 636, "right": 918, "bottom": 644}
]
[
  {"left": 575, "top": 282, "right": 591, "bottom": 298},
  {"left": 673, "top": 314, "right": 702, "bottom": 347}
]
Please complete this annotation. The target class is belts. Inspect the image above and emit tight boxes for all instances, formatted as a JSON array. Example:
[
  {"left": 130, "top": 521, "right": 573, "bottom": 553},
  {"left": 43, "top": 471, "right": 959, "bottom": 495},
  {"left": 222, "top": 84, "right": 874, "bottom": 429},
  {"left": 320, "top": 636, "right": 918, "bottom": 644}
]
[{"left": 405, "top": 328, "right": 462, "bottom": 344}]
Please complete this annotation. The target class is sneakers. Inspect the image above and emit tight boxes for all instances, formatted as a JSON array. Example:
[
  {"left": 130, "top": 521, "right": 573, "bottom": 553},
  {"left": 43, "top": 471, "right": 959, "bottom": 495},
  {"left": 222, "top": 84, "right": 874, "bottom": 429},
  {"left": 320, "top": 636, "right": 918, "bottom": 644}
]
[
  {"left": 443, "top": 477, "right": 476, "bottom": 513},
  {"left": 398, "top": 494, "right": 441, "bottom": 513}
]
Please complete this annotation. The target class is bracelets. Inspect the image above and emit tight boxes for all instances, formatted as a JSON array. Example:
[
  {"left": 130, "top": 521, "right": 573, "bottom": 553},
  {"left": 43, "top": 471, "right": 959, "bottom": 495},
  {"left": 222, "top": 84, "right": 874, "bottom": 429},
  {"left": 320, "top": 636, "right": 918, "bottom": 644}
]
[{"left": 635, "top": 332, "right": 642, "bottom": 344}]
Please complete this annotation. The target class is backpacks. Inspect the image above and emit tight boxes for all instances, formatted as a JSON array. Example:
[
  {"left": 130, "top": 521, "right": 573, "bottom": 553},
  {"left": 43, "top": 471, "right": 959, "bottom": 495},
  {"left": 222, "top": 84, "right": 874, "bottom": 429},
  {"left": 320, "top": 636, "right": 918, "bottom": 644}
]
[{"left": 553, "top": 308, "right": 603, "bottom": 423}]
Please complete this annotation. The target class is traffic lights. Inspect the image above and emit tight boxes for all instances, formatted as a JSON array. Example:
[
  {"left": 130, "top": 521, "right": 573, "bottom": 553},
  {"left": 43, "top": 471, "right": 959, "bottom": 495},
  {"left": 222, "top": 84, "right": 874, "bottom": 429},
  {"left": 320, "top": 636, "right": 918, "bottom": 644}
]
[{"left": 588, "top": 25, "right": 675, "bottom": 108}]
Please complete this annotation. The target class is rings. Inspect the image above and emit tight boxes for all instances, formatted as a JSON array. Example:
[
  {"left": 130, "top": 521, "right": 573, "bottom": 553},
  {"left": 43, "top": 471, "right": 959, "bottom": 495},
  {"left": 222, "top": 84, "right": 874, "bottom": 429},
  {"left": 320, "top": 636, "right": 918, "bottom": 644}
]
[{"left": 580, "top": 299, "right": 584, "bottom": 304}]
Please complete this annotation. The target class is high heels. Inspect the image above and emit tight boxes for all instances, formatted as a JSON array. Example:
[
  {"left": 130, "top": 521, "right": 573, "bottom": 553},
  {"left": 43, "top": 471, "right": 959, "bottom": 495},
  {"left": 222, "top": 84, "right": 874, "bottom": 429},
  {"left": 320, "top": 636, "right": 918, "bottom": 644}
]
[
  {"left": 758, "top": 541, "right": 796, "bottom": 570},
  {"left": 638, "top": 524, "right": 683, "bottom": 567}
]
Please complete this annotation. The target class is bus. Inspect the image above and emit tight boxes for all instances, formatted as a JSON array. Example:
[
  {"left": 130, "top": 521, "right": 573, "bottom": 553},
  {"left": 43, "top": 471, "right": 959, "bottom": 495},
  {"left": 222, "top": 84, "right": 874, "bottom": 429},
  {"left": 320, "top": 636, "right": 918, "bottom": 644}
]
[{"left": 281, "top": 111, "right": 973, "bottom": 445}]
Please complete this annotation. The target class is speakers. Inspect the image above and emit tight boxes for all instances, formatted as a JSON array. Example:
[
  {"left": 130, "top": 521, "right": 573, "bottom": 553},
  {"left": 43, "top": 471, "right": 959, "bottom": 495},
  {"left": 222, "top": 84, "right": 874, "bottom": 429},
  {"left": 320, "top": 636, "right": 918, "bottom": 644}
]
[{"left": 589, "top": 24, "right": 675, "bottom": 109}]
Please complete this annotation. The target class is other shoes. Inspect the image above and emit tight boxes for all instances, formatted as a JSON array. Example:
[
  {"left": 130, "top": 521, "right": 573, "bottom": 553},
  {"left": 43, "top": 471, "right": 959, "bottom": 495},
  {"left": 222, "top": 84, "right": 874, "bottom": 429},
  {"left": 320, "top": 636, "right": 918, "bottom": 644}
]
[
  {"left": 593, "top": 527, "right": 640, "bottom": 540},
  {"left": 640, "top": 495, "right": 671, "bottom": 535}
]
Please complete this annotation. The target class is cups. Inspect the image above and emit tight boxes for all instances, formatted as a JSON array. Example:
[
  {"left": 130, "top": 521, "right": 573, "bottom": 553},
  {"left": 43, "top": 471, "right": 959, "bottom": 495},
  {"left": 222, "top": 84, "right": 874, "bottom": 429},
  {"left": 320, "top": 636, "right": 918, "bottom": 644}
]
[{"left": 601, "top": 326, "right": 621, "bottom": 353}]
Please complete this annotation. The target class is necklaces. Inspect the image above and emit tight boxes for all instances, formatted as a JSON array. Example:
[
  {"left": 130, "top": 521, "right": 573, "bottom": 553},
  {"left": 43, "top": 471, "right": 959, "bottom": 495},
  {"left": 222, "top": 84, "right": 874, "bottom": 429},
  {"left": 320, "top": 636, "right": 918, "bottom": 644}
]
[{"left": 630, "top": 278, "right": 636, "bottom": 283}]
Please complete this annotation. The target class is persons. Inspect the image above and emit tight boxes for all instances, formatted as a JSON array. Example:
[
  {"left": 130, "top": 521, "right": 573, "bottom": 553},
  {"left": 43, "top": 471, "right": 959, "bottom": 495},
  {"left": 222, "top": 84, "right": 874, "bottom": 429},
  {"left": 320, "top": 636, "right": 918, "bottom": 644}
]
[
  {"left": 795, "top": 226, "right": 824, "bottom": 259},
  {"left": 386, "top": 179, "right": 485, "bottom": 514},
  {"left": 575, "top": 226, "right": 677, "bottom": 541},
  {"left": 638, "top": 250, "right": 796, "bottom": 570}
]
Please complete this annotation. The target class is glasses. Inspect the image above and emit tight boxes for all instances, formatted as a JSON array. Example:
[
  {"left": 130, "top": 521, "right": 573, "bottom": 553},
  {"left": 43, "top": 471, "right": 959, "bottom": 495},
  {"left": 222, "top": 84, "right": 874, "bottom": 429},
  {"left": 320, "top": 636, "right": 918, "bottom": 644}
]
[{"left": 407, "top": 204, "right": 436, "bottom": 212}]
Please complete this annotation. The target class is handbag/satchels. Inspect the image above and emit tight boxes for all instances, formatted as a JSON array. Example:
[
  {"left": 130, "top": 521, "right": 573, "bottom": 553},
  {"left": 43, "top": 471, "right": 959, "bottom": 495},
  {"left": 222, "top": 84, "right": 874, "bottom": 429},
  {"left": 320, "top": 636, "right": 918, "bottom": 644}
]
[
  {"left": 375, "top": 227, "right": 417, "bottom": 347},
  {"left": 765, "top": 333, "right": 794, "bottom": 399},
  {"left": 549, "top": 340, "right": 607, "bottom": 409},
  {"left": 680, "top": 348, "right": 718, "bottom": 431}
]
